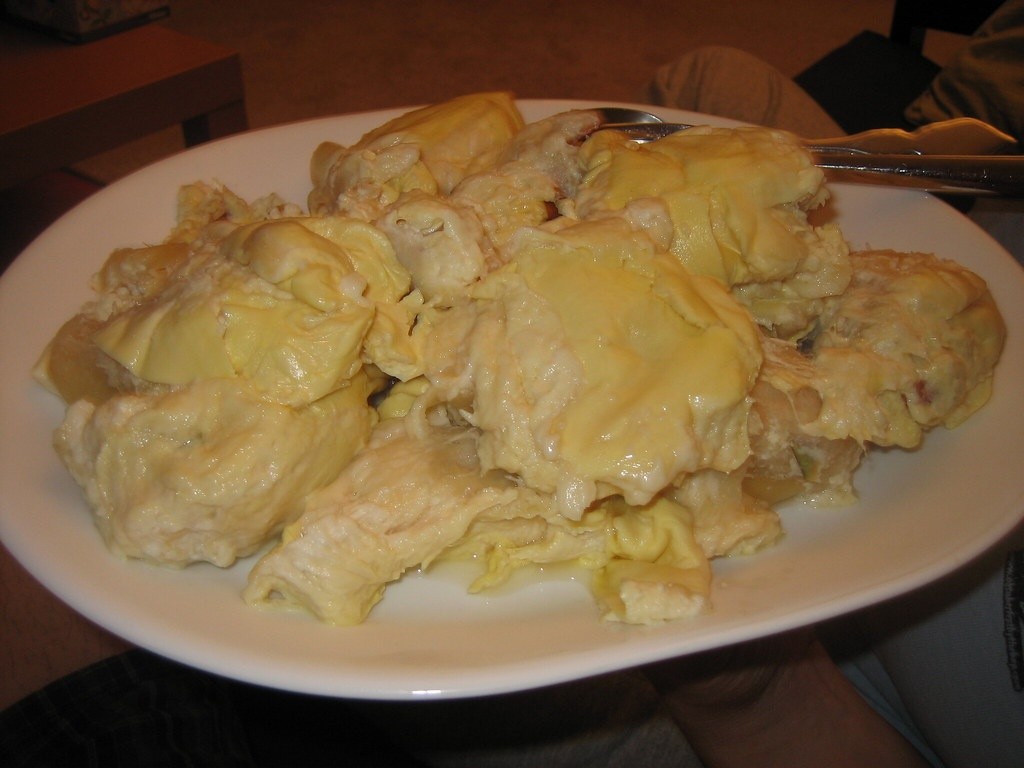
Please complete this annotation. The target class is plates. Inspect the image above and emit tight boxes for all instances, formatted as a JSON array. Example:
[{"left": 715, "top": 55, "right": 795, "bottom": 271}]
[{"left": 0, "top": 98, "right": 1024, "bottom": 700}]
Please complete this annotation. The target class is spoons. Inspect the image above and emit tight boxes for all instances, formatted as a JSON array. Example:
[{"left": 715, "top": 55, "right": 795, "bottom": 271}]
[{"left": 581, "top": 108, "right": 923, "bottom": 157}]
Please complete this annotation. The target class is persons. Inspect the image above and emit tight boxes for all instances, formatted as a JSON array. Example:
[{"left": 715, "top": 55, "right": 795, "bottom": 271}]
[{"left": 1, "top": 45, "right": 1024, "bottom": 768}]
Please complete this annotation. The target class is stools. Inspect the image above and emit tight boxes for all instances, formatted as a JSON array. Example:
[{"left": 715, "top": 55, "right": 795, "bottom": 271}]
[{"left": 0, "top": 23, "right": 251, "bottom": 213}]
